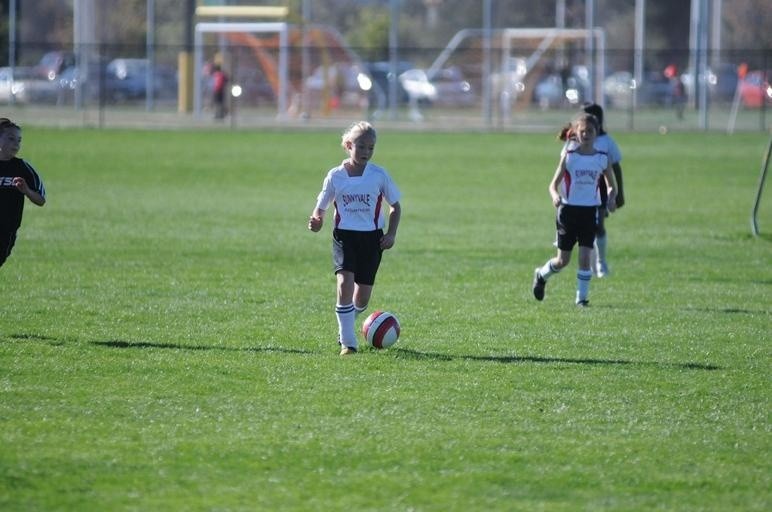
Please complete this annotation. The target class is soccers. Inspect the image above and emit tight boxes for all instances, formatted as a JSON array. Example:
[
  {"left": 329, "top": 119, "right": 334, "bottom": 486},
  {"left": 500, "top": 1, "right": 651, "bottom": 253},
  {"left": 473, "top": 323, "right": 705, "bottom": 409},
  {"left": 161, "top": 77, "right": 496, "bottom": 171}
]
[{"left": 363, "top": 311, "right": 401, "bottom": 349}]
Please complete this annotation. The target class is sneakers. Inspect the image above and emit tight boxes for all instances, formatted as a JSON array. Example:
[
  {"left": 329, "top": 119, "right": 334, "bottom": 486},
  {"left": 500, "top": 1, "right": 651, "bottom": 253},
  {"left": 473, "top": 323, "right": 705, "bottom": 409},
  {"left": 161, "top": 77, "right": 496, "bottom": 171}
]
[
  {"left": 533, "top": 266, "right": 547, "bottom": 300},
  {"left": 591, "top": 257, "right": 608, "bottom": 279},
  {"left": 575, "top": 297, "right": 589, "bottom": 307},
  {"left": 338, "top": 330, "right": 358, "bottom": 355}
]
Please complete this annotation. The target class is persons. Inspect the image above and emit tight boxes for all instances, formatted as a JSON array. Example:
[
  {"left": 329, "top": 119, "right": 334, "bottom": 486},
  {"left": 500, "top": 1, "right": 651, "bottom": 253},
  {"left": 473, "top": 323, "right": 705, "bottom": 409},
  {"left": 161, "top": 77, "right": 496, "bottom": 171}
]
[
  {"left": 559, "top": 100, "right": 626, "bottom": 281},
  {"left": 307, "top": 119, "right": 404, "bottom": 357},
  {"left": 0, "top": 115, "right": 47, "bottom": 269},
  {"left": 211, "top": 65, "right": 230, "bottom": 121},
  {"left": 530, "top": 110, "right": 618, "bottom": 309}
]
